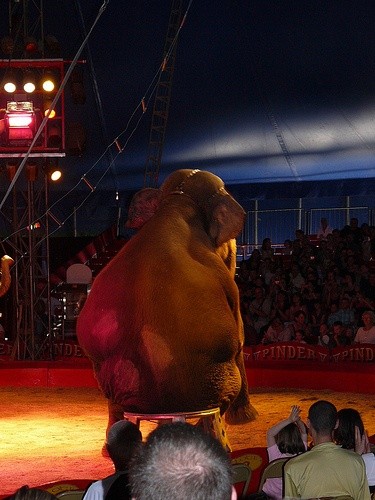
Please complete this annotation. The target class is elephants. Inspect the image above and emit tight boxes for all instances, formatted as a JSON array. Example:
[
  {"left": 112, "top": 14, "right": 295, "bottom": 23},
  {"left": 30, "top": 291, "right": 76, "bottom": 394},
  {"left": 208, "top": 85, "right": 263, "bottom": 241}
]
[{"left": 75, "top": 169, "right": 259, "bottom": 458}]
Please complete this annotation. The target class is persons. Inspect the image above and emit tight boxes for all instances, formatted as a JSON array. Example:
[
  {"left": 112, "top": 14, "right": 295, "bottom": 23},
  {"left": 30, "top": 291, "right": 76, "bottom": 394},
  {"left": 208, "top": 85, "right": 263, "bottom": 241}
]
[
  {"left": 128, "top": 421, "right": 237, "bottom": 500},
  {"left": 263, "top": 400, "right": 375, "bottom": 500},
  {"left": 80, "top": 420, "right": 144, "bottom": 500},
  {"left": 237, "top": 213, "right": 375, "bottom": 348}
]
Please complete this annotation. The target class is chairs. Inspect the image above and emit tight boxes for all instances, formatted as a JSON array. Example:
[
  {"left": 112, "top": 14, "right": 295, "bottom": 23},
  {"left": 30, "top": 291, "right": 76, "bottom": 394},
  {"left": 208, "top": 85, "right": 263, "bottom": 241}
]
[
  {"left": 258, "top": 457, "right": 288, "bottom": 492},
  {"left": 230, "top": 463, "right": 252, "bottom": 496}
]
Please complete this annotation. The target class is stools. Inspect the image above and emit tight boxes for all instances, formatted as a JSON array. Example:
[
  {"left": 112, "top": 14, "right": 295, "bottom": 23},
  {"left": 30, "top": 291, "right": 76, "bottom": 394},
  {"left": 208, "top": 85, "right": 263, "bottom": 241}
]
[{"left": 123, "top": 407, "right": 233, "bottom": 451}]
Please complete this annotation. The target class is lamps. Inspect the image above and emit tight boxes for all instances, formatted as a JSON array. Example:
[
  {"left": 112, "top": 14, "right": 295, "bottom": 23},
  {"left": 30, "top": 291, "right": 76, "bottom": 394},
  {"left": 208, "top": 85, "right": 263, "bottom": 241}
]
[{"left": 4, "top": 101, "right": 37, "bottom": 139}]
